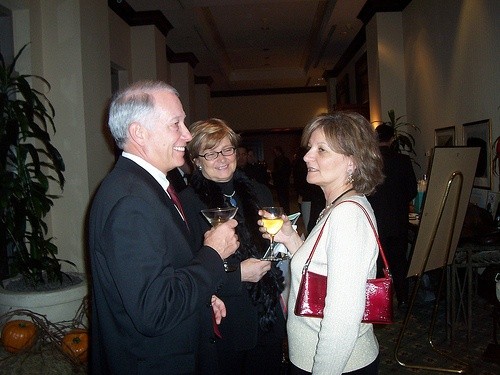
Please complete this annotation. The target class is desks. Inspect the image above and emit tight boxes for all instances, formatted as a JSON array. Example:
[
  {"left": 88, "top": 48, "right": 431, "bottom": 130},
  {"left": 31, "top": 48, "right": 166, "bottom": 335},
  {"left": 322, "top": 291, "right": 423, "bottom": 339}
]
[{"left": 407, "top": 221, "right": 500, "bottom": 344}]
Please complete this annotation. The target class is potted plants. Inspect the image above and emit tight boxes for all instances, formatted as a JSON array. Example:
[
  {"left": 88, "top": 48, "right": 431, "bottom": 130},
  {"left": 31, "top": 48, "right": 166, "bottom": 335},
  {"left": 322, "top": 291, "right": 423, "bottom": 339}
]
[{"left": 0, "top": 37, "right": 92, "bottom": 330}]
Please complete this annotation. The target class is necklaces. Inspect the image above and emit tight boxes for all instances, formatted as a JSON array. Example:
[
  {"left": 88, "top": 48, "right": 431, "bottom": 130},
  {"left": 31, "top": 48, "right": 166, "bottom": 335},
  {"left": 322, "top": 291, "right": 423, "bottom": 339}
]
[{"left": 315, "top": 188, "right": 354, "bottom": 224}]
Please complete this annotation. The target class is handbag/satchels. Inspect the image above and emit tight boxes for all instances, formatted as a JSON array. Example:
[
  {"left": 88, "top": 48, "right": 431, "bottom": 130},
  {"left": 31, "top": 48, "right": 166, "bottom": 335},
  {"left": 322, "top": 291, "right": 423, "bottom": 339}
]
[{"left": 293, "top": 200, "right": 394, "bottom": 326}]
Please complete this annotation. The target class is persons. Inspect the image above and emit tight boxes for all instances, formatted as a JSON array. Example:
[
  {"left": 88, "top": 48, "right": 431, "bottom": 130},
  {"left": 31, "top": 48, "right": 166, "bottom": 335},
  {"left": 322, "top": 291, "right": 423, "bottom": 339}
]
[
  {"left": 179, "top": 118, "right": 290, "bottom": 375},
  {"left": 273, "top": 145, "right": 291, "bottom": 215},
  {"left": 258, "top": 112, "right": 386, "bottom": 375},
  {"left": 292, "top": 151, "right": 306, "bottom": 204},
  {"left": 235, "top": 145, "right": 271, "bottom": 183},
  {"left": 367, "top": 124, "right": 418, "bottom": 305},
  {"left": 88, "top": 78, "right": 241, "bottom": 375},
  {"left": 167, "top": 162, "right": 193, "bottom": 192}
]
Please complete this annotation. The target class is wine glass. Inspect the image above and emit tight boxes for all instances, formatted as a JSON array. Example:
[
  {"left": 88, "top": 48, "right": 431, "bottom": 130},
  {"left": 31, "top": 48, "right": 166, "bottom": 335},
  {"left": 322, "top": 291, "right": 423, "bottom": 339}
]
[
  {"left": 201, "top": 206, "right": 240, "bottom": 273},
  {"left": 262, "top": 206, "right": 285, "bottom": 262}
]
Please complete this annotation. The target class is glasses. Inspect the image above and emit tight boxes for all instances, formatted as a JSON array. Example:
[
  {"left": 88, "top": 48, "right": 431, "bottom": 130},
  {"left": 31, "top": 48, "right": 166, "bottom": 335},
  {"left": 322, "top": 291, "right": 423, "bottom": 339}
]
[{"left": 198, "top": 147, "right": 237, "bottom": 161}]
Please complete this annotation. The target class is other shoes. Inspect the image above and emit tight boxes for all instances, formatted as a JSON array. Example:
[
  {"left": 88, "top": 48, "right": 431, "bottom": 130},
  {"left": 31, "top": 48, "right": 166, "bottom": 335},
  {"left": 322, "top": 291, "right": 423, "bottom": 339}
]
[{"left": 399, "top": 297, "right": 414, "bottom": 313}]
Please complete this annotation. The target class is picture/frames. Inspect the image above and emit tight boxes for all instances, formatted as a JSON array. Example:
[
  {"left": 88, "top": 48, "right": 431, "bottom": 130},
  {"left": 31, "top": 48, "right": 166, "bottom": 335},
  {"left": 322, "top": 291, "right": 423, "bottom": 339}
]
[
  {"left": 432, "top": 124, "right": 457, "bottom": 148},
  {"left": 459, "top": 118, "right": 493, "bottom": 191},
  {"left": 335, "top": 50, "right": 369, "bottom": 105}
]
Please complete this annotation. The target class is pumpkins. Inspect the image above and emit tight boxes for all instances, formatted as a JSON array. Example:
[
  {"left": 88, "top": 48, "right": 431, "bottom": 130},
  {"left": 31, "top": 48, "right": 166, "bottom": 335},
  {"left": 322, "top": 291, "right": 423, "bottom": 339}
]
[
  {"left": 1, "top": 319, "right": 36, "bottom": 353},
  {"left": 61, "top": 329, "right": 87, "bottom": 364}
]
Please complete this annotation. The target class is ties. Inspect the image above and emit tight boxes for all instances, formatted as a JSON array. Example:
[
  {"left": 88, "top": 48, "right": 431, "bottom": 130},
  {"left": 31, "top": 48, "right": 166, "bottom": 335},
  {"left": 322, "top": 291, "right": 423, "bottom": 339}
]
[{"left": 167, "top": 181, "right": 223, "bottom": 340}]
